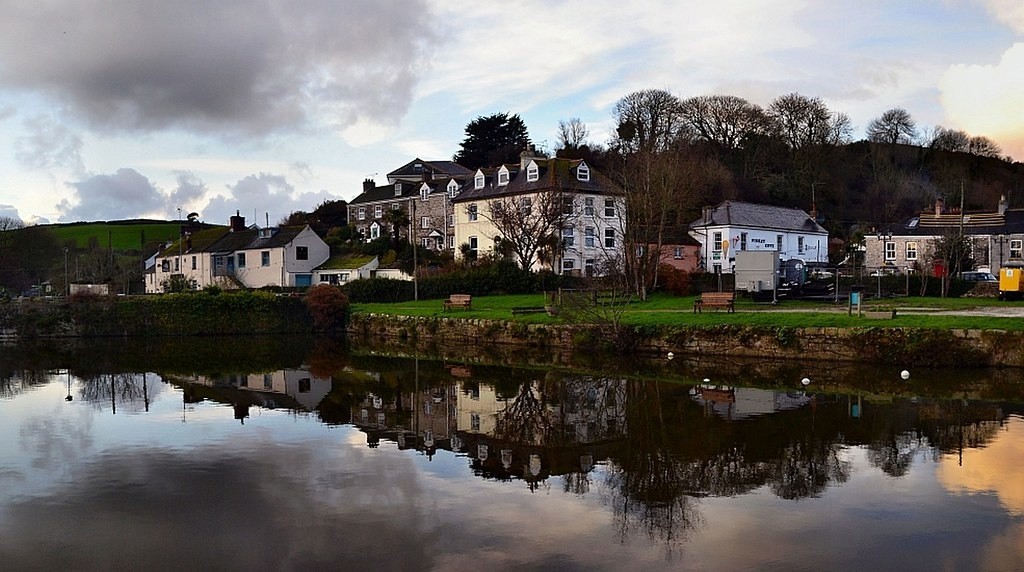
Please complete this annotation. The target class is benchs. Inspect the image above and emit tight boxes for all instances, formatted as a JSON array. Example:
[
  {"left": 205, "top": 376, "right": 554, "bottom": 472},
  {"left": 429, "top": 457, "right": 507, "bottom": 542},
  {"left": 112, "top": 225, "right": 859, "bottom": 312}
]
[
  {"left": 693, "top": 291, "right": 736, "bottom": 315},
  {"left": 442, "top": 294, "right": 472, "bottom": 312}
]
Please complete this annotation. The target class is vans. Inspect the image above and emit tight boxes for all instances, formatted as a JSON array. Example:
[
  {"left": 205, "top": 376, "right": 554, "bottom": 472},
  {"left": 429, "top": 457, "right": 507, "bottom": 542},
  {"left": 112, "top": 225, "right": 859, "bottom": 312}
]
[{"left": 956, "top": 270, "right": 997, "bottom": 282}]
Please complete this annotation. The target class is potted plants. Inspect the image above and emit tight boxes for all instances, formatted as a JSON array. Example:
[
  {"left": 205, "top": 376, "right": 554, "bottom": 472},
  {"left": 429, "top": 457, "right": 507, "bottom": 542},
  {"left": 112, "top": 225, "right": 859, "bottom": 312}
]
[{"left": 865, "top": 302, "right": 897, "bottom": 320}]
